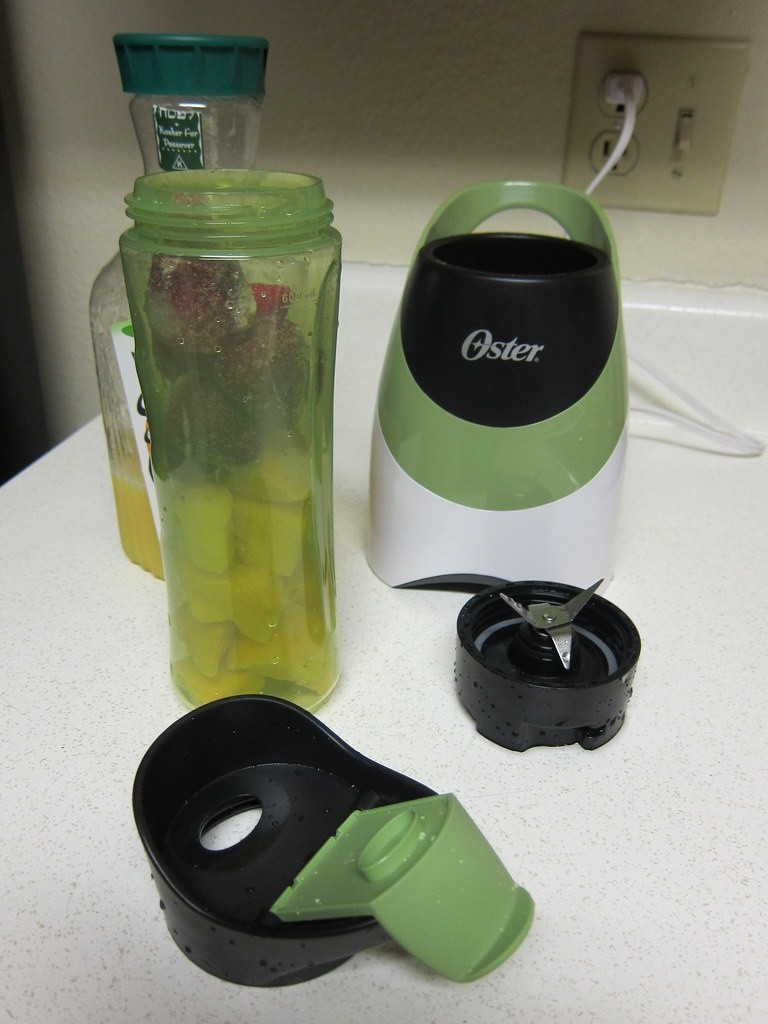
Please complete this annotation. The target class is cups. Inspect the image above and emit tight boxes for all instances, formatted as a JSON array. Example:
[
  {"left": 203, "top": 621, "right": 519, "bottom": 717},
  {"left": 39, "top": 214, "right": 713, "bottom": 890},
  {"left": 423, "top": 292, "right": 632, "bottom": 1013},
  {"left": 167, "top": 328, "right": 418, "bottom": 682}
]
[{"left": 118, "top": 165, "right": 341, "bottom": 720}]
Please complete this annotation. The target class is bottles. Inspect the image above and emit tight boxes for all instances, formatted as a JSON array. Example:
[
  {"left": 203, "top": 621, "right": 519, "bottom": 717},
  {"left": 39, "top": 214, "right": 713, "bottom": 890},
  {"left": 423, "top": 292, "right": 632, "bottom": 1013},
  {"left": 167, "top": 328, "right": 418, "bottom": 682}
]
[{"left": 88, "top": 32, "right": 271, "bottom": 582}]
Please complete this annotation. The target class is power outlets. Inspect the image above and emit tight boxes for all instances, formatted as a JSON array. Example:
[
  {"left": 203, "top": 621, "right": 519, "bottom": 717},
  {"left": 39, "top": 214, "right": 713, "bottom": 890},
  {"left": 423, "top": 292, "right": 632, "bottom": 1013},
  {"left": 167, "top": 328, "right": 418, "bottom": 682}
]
[{"left": 558, "top": 31, "right": 751, "bottom": 216}]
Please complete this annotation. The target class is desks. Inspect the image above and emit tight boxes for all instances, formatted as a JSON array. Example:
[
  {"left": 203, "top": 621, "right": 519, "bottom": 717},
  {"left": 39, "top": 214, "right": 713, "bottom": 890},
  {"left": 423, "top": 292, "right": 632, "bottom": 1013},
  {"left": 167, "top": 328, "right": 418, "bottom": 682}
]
[{"left": 0, "top": 261, "right": 768, "bottom": 1023}]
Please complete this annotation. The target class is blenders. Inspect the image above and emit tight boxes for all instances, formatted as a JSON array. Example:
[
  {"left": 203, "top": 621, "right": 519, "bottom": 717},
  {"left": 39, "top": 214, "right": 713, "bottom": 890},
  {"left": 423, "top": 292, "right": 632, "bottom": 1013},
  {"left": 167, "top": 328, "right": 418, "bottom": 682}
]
[{"left": 363, "top": 180, "right": 631, "bottom": 594}]
